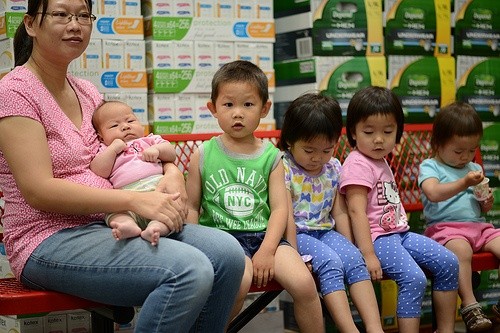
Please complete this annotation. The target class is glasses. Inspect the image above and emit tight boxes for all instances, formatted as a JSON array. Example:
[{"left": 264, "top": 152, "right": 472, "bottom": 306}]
[{"left": 35, "top": 10, "right": 96, "bottom": 25}]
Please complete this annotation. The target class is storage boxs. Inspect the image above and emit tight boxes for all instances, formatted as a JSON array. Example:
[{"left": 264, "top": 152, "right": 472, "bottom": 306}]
[
  {"left": 273, "top": 0, "right": 500, "bottom": 333},
  {"left": 0, "top": 0, "right": 149, "bottom": 333},
  {"left": 143, "top": 2, "right": 279, "bottom": 333}
]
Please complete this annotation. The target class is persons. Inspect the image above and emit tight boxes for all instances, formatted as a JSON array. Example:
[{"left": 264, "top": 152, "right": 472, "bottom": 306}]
[
  {"left": 280, "top": 94, "right": 384, "bottom": 333},
  {"left": 90, "top": 101, "right": 176, "bottom": 245},
  {"left": 1, "top": 0, "right": 246, "bottom": 333},
  {"left": 418, "top": 102, "right": 500, "bottom": 333},
  {"left": 185, "top": 60, "right": 324, "bottom": 333},
  {"left": 340, "top": 87, "right": 459, "bottom": 333}
]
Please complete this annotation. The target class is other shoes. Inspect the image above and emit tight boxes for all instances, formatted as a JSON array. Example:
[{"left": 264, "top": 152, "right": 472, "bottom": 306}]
[
  {"left": 460, "top": 302, "right": 493, "bottom": 332},
  {"left": 492, "top": 302, "right": 500, "bottom": 315}
]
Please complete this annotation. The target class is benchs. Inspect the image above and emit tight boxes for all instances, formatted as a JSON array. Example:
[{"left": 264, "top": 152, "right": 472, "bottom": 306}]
[{"left": 0, "top": 122, "right": 500, "bottom": 333}]
